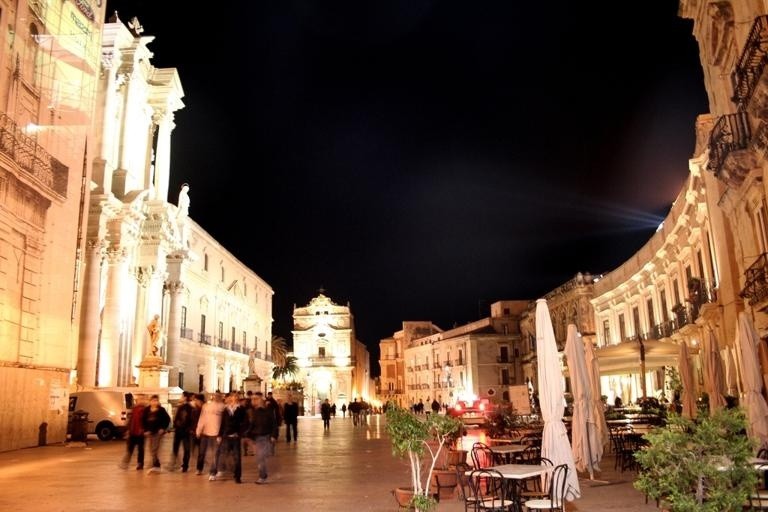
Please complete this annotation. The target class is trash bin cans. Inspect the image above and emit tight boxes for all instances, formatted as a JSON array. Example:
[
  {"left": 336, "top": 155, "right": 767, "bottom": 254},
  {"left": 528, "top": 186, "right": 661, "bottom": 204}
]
[{"left": 70, "top": 409, "right": 89, "bottom": 442}]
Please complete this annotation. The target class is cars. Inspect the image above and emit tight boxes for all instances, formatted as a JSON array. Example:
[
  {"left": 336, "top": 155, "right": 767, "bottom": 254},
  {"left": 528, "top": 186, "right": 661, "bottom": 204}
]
[{"left": 448, "top": 397, "right": 513, "bottom": 426}]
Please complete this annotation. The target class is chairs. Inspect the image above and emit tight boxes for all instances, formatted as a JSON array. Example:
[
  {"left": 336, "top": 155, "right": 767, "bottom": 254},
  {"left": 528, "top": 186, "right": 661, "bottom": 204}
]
[
  {"left": 473, "top": 413, "right": 543, "bottom": 468},
  {"left": 457, "top": 461, "right": 499, "bottom": 512},
  {"left": 616, "top": 427, "right": 642, "bottom": 473},
  {"left": 562, "top": 420, "right": 573, "bottom": 444},
  {"left": 519, "top": 457, "right": 554, "bottom": 501},
  {"left": 470, "top": 467, "right": 515, "bottom": 512},
  {"left": 469, "top": 447, "right": 508, "bottom": 499},
  {"left": 524, "top": 463, "right": 568, "bottom": 511},
  {"left": 608, "top": 428, "right": 630, "bottom": 472},
  {"left": 745, "top": 446, "right": 767, "bottom": 510},
  {"left": 605, "top": 412, "right": 666, "bottom": 452}
]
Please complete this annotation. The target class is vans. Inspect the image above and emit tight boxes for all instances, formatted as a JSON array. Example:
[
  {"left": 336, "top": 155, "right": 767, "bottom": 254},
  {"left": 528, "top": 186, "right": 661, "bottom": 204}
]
[{"left": 67, "top": 390, "right": 133, "bottom": 442}]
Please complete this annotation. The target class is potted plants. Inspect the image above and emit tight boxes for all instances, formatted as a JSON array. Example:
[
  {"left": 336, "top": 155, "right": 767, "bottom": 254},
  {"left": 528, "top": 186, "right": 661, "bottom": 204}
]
[
  {"left": 439, "top": 418, "right": 469, "bottom": 470},
  {"left": 383, "top": 401, "right": 431, "bottom": 511},
  {"left": 426, "top": 416, "right": 462, "bottom": 501}
]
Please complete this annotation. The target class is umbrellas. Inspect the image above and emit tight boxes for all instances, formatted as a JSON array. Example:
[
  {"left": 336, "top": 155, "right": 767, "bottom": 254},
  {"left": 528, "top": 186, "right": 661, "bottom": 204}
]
[
  {"left": 583, "top": 335, "right": 609, "bottom": 459},
  {"left": 704, "top": 328, "right": 728, "bottom": 416},
  {"left": 563, "top": 323, "right": 603, "bottom": 481},
  {"left": 532, "top": 298, "right": 582, "bottom": 512},
  {"left": 733, "top": 312, "right": 768, "bottom": 453},
  {"left": 677, "top": 338, "right": 698, "bottom": 420},
  {"left": 724, "top": 344, "right": 739, "bottom": 398}
]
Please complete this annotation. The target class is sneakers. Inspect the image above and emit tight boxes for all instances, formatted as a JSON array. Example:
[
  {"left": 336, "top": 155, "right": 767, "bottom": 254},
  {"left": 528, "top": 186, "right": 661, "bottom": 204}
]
[
  {"left": 255, "top": 478, "right": 264, "bottom": 484},
  {"left": 120, "top": 463, "right": 225, "bottom": 476},
  {"left": 209, "top": 473, "right": 216, "bottom": 480},
  {"left": 232, "top": 476, "right": 242, "bottom": 484}
]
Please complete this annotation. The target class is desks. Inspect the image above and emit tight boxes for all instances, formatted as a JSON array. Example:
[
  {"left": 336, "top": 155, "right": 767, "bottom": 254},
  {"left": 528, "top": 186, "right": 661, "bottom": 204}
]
[
  {"left": 464, "top": 465, "right": 554, "bottom": 510},
  {"left": 611, "top": 429, "right": 659, "bottom": 450}
]
